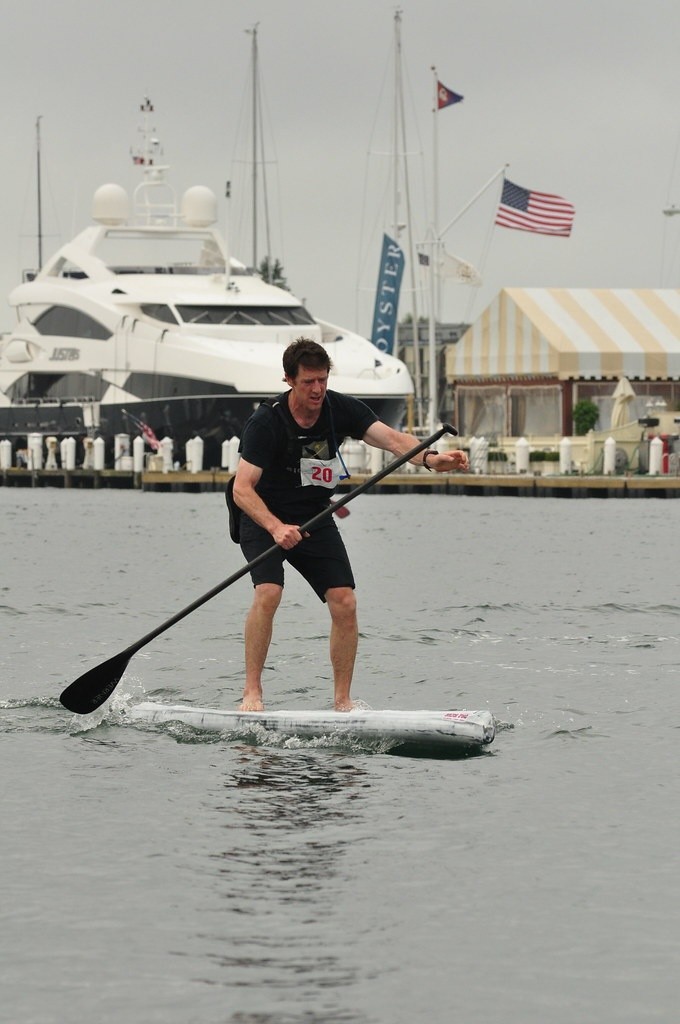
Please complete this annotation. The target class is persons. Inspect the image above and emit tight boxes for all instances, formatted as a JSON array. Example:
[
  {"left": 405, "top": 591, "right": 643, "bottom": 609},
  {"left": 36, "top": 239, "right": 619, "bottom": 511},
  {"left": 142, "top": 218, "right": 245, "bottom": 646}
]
[
  {"left": 228, "top": 338, "right": 471, "bottom": 716},
  {"left": 610, "top": 375, "right": 635, "bottom": 430}
]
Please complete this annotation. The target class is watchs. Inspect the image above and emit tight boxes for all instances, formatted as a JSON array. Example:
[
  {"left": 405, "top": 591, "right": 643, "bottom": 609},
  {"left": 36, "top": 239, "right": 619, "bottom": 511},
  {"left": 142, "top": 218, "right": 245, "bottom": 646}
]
[{"left": 423, "top": 450, "right": 438, "bottom": 473}]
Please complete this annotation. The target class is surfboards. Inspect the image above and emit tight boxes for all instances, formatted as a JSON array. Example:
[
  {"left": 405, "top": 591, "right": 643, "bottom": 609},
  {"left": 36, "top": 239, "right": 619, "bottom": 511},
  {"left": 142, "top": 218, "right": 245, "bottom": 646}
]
[{"left": 134, "top": 700, "right": 495, "bottom": 757}]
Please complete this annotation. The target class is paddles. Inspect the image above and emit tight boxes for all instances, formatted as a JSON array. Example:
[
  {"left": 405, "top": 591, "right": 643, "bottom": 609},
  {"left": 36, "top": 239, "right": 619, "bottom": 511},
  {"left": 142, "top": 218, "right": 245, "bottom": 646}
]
[{"left": 59, "top": 423, "right": 458, "bottom": 716}]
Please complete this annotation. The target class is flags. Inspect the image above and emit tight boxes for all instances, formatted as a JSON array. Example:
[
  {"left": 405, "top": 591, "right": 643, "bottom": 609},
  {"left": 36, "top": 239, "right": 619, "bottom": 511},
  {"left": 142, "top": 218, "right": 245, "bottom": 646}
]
[
  {"left": 436, "top": 80, "right": 464, "bottom": 110},
  {"left": 494, "top": 178, "right": 575, "bottom": 237},
  {"left": 122, "top": 410, "right": 163, "bottom": 450}
]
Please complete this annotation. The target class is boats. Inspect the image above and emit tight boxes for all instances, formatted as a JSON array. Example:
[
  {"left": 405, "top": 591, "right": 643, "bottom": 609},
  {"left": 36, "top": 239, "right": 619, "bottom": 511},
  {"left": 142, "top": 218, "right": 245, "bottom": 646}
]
[{"left": 1, "top": 97, "right": 423, "bottom": 470}]
